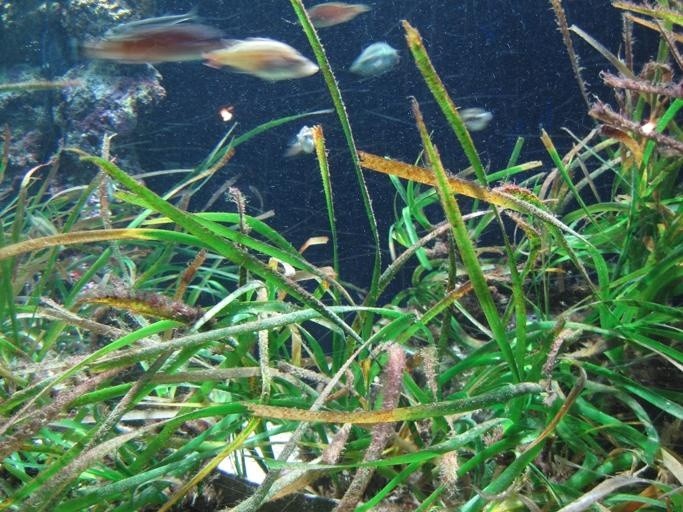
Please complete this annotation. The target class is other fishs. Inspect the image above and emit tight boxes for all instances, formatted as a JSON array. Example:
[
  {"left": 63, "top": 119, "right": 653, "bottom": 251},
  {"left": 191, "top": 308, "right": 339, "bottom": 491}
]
[{"left": 88, "top": 287, "right": 197, "bottom": 322}]
